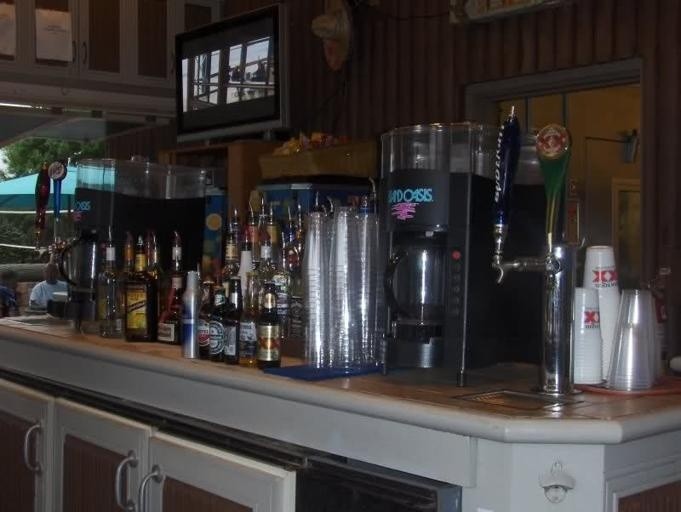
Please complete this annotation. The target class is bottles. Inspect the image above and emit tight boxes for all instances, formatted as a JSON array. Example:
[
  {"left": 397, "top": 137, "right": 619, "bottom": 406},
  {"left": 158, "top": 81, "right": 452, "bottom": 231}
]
[{"left": 93, "top": 199, "right": 308, "bottom": 371}]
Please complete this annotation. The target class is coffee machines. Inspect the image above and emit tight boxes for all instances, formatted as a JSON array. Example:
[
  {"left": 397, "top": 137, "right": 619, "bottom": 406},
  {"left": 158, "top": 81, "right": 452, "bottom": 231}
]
[
  {"left": 375, "top": 118, "right": 570, "bottom": 392},
  {"left": 57, "top": 158, "right": 207, "bottom": 321}
]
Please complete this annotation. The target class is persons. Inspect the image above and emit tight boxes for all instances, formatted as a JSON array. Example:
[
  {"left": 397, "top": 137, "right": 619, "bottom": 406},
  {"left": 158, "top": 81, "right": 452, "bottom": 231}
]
[
  {"left": 247, "top": 60, "right": 265, "bottom": 95},
  {"left": 29, "top": 264, "right": 68, "bottom": 310},
  {"left": 0, "top": 270, "right": 20, "bottom": 316},
  {"left": 232, "top": 66, "right": 246, "bottom": 96}
]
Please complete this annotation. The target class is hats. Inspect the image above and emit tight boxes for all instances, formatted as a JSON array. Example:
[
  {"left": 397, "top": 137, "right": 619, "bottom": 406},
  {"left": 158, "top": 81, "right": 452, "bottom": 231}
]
[{"left": 312, "top": 0, "right": 350, "bottom": 71}]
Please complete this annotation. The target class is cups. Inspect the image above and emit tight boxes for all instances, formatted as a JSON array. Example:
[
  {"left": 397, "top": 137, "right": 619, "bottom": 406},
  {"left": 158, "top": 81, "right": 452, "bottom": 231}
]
[
  {"left": 300, "top": 205, "right": 392, "bottom": 372},
  {"left": 568, "top": 242, "right": 664, "bottom": 393}
]
